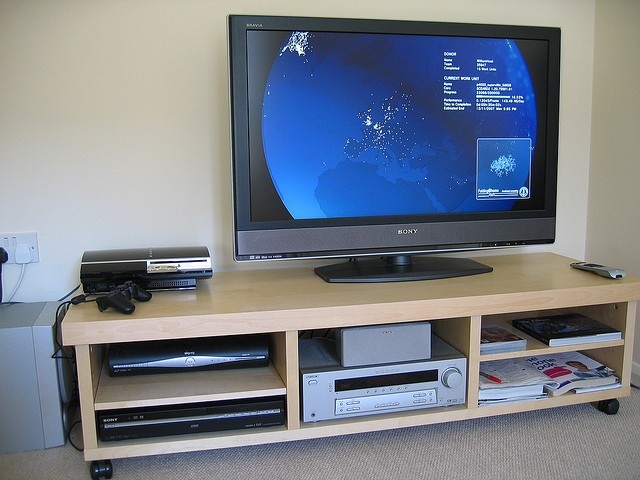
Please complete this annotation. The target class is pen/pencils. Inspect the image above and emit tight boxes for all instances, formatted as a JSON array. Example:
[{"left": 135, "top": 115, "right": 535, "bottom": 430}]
[{"left": 479, "top": 371, "right": 501, "bottom": 383}]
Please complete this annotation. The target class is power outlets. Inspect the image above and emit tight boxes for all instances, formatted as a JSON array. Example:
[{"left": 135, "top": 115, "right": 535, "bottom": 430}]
[{"left": 1, "top": 232, "right": 39, "bottom": 264}]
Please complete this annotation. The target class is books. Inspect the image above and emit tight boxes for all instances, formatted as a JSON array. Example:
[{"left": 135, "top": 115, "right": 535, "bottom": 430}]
[
  {"left": 528, "top": 352, "right": 623, "bottom": 398},
  {"left": 478, "top": 360, "right": 559, "bottom": 406},
  {"left": 512, "top": 312, "right": 622, "bottom": 348},
  {"left": 480, "top": 328, "right": 527, "bottom": 354}
]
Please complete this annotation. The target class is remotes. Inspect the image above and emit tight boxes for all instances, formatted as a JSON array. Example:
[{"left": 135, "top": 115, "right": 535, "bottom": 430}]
[{"left": 570, "top": 262, "right": 625, "bottom": 279}]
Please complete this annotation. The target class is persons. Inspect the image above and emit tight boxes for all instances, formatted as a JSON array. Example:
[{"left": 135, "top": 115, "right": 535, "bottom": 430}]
[{"left": 566, "top": 361, "right": 615, "bottom": 380}]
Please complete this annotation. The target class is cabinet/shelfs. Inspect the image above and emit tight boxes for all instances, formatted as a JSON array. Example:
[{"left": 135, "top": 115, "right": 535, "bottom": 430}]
[{"left": 61, "top": 252, "right": 640, "bottom": 480}]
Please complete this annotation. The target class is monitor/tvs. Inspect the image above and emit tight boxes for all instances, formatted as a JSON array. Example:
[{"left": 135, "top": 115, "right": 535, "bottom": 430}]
[{"left": 229, "top": 15, "right": 561, "bottom": 280}]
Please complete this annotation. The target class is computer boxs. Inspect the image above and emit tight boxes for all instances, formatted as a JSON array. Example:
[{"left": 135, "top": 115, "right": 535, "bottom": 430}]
[{"left": 1, "top": 300, "right": 66, "bottom": 456}]
